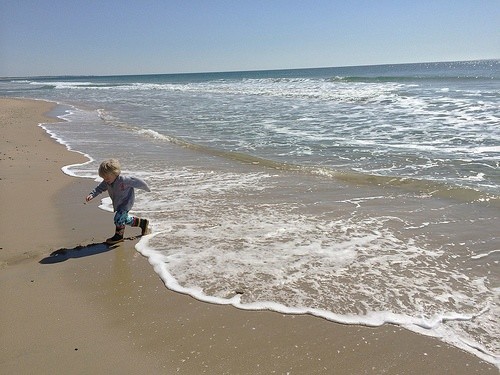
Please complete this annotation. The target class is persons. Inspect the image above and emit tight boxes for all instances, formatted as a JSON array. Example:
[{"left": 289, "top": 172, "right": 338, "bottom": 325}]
[{"left": 85, "top": 159, "right": 152, "bottom": 244}]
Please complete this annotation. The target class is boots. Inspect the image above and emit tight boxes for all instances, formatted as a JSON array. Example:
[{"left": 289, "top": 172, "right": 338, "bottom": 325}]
[
  {"left": 106, "top": 225, "right": 125, "bottom": 243},
  {"left": 132, "top": 217, "right": 150, "bottom": 235}
]
